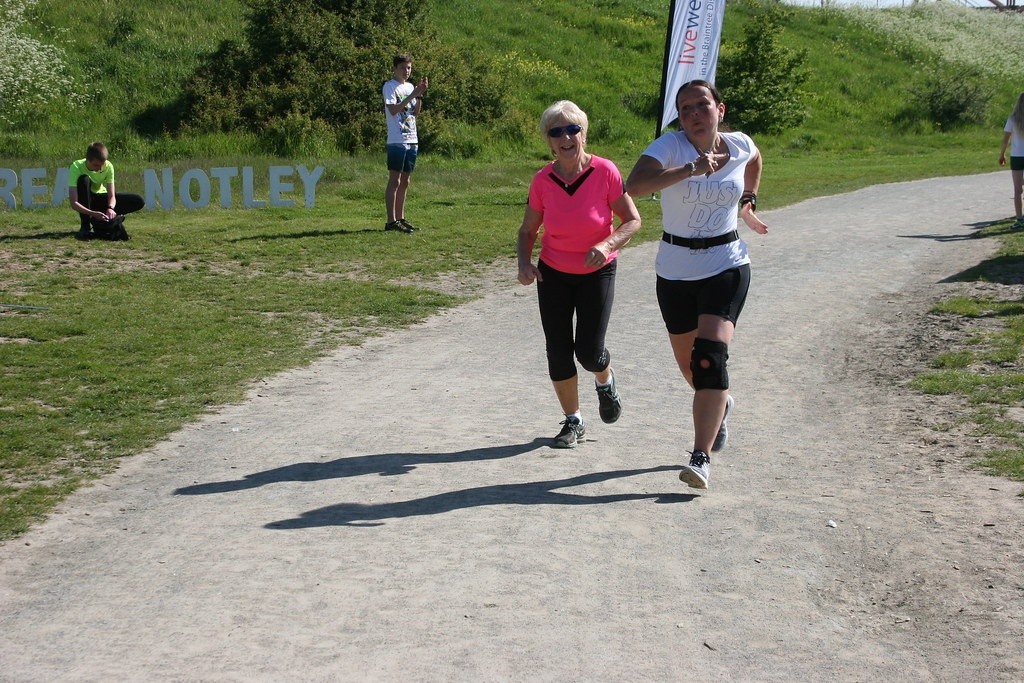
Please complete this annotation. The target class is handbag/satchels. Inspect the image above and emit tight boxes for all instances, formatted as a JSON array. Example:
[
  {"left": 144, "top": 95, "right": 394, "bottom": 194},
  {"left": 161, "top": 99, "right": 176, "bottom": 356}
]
[{"left": 91, "top": 215, "right": 130, "bottom": 241}]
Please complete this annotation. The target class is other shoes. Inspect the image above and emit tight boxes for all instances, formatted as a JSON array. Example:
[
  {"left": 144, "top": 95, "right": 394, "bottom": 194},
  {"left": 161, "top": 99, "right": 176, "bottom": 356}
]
[
  {"left": 79, "top": 228, "right": 90, "bottom": 240},
  {"left": 1011, "top": 221, "right": 1024, "bottom": 230}
]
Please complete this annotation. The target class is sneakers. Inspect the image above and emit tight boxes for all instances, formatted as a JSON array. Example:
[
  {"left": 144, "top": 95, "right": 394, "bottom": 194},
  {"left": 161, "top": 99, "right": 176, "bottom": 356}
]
[
  {"left": 712, "top": 395, "right": 734, "bottom": 452},
  {"left": 554, "top": 411, "right": 585, "bottom": 447},
  {"left": 385, "top": 221, "right": 413, "bottom": 233},
  {"left": 396, "top": 219, "right": 420, "bottom": 230},
  {"left": 594, "top": 367, "right": 621, "bottom": 423},
  {"left": 680, "top": 450, "right": 711, "bottom": 490}
]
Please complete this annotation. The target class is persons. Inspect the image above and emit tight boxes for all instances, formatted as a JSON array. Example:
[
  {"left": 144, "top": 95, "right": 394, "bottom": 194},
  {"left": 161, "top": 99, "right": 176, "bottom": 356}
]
[
  {"left": 67, "top": 143, "right": 146, "bottom": 240},
  {"left": 999, "top": 92, "right": 1024, "bottom": 228},
  {"left": 382, "top": 55, "right": 429, "bottom": 232},
  {"left": 517, "top": 100, "right": 642, "bottom": 449},
  {"left": 627, "top": 80, "right": 768, "bottom": 491}
]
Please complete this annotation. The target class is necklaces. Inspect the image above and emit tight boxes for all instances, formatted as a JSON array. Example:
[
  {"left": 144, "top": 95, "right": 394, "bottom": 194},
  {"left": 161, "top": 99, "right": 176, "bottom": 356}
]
[{"left": 556, "top": 160, "right": 578, "bottom": 188}]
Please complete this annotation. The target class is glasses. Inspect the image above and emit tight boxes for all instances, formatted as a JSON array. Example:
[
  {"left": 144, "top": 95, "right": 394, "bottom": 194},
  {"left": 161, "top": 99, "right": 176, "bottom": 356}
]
[{"left": 547, "top": 124, "right": 582, "bottom": 138}]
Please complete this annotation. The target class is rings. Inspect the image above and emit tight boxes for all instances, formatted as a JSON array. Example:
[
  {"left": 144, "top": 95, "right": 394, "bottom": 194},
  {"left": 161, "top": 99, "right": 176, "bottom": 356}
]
[{"left": 713, "top": 161, "right": 717, "bottom": 167}]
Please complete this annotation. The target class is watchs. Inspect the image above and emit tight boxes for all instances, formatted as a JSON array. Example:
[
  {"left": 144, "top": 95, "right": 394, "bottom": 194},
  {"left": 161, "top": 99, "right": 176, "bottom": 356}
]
[
  {"left": 417, "top": 95, "right": 424, "bottom": 100},
  {"left": 741, "top": 199, "right": 756, "bottom": 212}
]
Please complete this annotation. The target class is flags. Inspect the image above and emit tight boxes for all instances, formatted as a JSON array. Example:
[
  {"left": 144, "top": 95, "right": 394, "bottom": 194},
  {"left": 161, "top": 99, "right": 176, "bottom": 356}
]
[{"left": 661, "top": 1, "right": 726, "bottom": 136}]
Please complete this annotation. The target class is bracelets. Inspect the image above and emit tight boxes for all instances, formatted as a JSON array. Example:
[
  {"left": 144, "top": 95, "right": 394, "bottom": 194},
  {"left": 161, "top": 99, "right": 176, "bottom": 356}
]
[
  {"left": 739, "top": 190, "right": 756, "bottom": 203},
  {"left": 108, "top": 206, "right": 114, "bottom": 209},
  {"left": 685, "top": 161, "right": 696, "bottom": 178}
]
